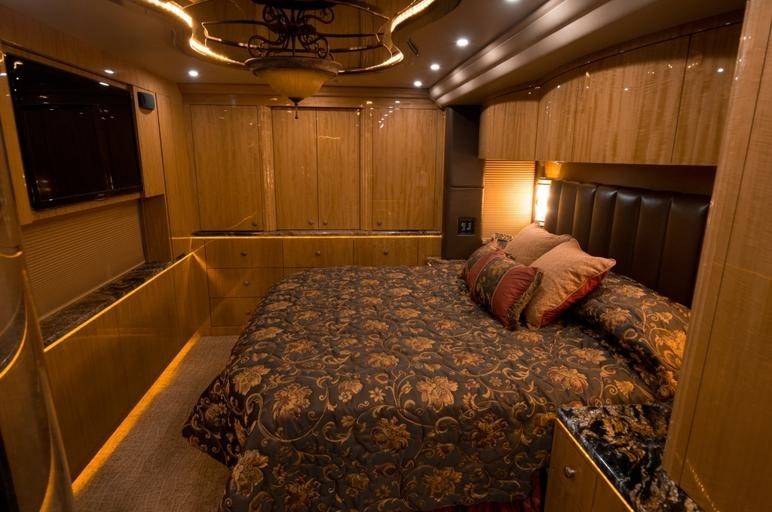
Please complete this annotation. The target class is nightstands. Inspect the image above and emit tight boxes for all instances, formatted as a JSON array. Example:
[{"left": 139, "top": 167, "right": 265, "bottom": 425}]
[{"left": 548, "top": 405, "right": 704, "bottom": 512}]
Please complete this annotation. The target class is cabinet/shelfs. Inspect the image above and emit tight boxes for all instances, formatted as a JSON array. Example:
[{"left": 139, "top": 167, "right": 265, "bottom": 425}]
[
  {"left": 361, "top": 108, "right": 445, "bottom": 232},
  {"left": 662, "top": 0, "right": 772, "bottom": 511},
  {"left": 354, "top": 236, "right": 443, "bottom": 266},
  {"left": 282, "top": 236, "right": 355, "bottom": 279},
  {"left": 205, "top": 239, "right": 281, "bottom": 337},
  {"left": 267, "top": 106, "right": 361, "bottom": 231},
  {"left": 189, "top": 104, "right": 267, "bottom": 232}
]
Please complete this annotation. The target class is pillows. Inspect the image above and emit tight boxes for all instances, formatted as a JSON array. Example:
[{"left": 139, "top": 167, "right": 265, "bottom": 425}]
[
  {"left": 461, "top": 235, "right": 545, "bottom": 329},
  {"left": 505, "top": 222, "right": 574, "bottom": 266},
  {"left": 526, "top": 237, "right": 616, "bottom": 331}
]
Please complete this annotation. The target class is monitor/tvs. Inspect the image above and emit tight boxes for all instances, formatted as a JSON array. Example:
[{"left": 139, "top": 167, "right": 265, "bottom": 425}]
[{"left": 3, "top": 51, "right": 144, "bottom": 212}]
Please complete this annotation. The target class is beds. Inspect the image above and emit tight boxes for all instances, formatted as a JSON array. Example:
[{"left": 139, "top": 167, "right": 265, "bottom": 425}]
[{"left": 181, "top": 262, "right": 694, "bottom": 512}]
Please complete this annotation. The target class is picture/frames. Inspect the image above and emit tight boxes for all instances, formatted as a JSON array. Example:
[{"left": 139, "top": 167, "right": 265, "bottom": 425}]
[{"left": 457, "top": 217, "right": 474, "bottom": 234}]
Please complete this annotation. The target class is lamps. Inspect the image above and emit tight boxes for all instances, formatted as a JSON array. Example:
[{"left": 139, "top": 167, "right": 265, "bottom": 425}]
[{"left": 244, "top": 0, "right": 342, "bottom": 121}]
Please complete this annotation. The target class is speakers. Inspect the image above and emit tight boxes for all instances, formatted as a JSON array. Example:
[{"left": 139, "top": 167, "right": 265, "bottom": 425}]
[{"left": 137, "top": 92, "right": 155, "bottom": 110}]
